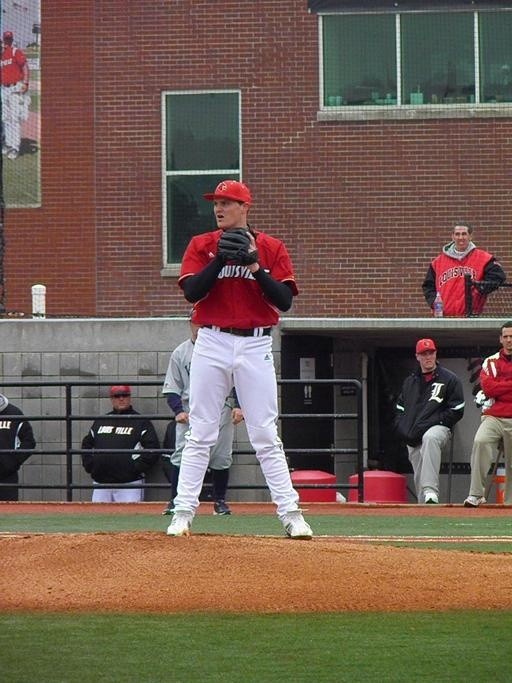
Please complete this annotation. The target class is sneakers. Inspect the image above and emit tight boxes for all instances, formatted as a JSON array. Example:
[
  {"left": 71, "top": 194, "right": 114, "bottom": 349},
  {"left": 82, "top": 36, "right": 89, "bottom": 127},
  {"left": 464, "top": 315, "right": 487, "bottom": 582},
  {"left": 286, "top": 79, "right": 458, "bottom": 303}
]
[
  {"left": 162, "top": 498, "right": 176, "bottom": 516},
  {"left": 212, "top": 499, "right": 233, "bottom": 516},
  {"left": 166, "top": 505, "right": 197, "bottom": 537},
  {"left": 424, "top": 491, "right": 439, "bottom": 505},
  {"left": 277, "top": 508, "right": 314, "bottom": 540},
  {"left": 463, "top": 494, "right": 488, "bottom": 508}
]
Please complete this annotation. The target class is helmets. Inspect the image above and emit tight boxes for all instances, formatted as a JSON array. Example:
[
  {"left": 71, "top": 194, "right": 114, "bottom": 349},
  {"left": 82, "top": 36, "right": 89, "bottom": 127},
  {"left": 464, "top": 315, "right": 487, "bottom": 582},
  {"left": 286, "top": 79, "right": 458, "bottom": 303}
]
[{"left": 186, "top": 307, "right": 196, "bottom": 323}]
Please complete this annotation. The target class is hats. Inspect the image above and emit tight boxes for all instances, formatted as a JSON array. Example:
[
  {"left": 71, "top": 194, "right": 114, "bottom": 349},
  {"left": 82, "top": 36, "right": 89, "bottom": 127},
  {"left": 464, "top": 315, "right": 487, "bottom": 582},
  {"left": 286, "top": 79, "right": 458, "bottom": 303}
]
[
  {"left": 201, "top": 179, "right": 253, "bottom": 206},
  {"left": 109, "top": 385, "right": 132, "bottom": 398},
  {"left": 415, "top": 338, "right": 440, "bottom": 354}
]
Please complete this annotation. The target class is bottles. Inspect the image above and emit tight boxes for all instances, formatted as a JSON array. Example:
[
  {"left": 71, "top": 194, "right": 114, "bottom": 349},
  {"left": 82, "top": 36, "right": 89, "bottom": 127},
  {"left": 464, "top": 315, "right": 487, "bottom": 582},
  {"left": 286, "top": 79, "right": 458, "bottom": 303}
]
[
  {"left": 432, "top": 290, "right": 443, "bottom": 317},
  {"left": 496, "top": 468, "right": 506, "bottom": 503}
]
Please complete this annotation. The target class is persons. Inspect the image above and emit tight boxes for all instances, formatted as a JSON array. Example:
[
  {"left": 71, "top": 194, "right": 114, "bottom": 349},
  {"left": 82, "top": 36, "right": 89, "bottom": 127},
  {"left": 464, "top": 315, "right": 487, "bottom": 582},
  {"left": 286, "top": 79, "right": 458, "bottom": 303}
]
[
  {"left": 167, "top": 182, "right": 313, "bottom": 538},
  {"left": 463, "top": 321, "right": 512, "bottom": 508},
  {"left": 393, "top": 338, "right": 466, "bottom": 504},
  {"left": 0, "top": 31, "right": 31, "bottom": 160},
  {"left": 422, "top": 221, "right": 506, "bottom": 317},
  {"left": 81, "top": 384, "right": 160, "bottom": 503},
  {"left": 162, "top": 422, "right": 215, "bottom": 501},
  {"left": 163, "top": 309, "right": 244, "bottom": 516},
  {"left": 0, "top": 394, "right": 36, "bottom": 502}
]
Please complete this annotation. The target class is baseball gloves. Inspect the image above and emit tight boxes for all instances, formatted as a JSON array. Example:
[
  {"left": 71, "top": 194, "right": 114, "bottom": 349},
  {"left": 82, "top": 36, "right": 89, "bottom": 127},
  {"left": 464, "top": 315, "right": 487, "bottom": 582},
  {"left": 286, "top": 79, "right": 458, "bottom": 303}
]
[{"left": 217, "top": 228, "right": 259, "bottom": 266}]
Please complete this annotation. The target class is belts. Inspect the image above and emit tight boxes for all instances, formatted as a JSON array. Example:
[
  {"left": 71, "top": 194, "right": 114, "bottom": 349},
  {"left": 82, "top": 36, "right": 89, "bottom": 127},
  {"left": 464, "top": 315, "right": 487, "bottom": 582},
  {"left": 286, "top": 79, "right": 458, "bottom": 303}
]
[{"left": 202, "top": 323, "right": 272, "bottom": 337}]
